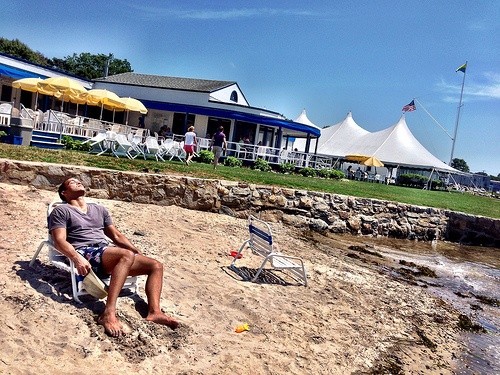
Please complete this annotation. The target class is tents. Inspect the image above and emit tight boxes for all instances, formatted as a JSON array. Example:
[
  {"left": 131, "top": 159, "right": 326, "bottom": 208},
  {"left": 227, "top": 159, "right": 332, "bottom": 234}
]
[{"left": 258, "top": 108, "right": 473, "bottom": 176}]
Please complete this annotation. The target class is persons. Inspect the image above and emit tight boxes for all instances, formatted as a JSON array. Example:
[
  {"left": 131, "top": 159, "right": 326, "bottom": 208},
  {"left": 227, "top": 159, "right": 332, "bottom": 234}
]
[
  {"left": 159, "top": 125, "right": 173, "bottom": 140},
  {"left": 240, "top": 137, "right": 272, "bottom": 160},
  {"left": 210, "top": 126, "right": 228, "bottom": 169},
  {"left": 48, "top": 177, "right": 181, "bottom": 338},
  {"left": 184, "top": 126, "right": 198, "bottom": 166}
]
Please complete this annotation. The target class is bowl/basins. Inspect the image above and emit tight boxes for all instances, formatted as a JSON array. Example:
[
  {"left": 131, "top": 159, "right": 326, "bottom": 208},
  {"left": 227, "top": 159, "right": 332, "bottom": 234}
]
[
  {"left": 82, "top": 273, "right": 108, "bottom": 299},
  {"left": 230, "top": 251, "right": 243, "bottom": 259}
]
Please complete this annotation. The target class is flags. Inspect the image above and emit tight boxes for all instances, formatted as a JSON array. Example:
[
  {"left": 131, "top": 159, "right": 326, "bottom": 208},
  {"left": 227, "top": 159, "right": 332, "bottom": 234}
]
[
  {"left": 402, "top": 101, "right": 415, "bottom": 111},
  {"left": 456, "top": 63, "right": 466, "bottom": 72}
]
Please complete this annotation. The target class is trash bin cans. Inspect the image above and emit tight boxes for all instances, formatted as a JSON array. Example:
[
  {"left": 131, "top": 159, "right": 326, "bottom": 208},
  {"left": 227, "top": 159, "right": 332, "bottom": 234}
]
[{"left": 9, "top": 125, "right": 23, "bottom": 144}]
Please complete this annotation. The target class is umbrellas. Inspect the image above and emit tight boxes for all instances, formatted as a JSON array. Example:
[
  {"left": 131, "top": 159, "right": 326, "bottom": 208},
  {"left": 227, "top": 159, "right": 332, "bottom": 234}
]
[{"left": 12, "top": 75, "right": 148, "bottom": 125}]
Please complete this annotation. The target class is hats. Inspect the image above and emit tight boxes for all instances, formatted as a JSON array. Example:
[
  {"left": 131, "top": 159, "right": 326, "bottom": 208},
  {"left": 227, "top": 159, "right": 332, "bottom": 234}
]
[{"left": 82, "top": 268, "right": 109, "bottom": 299}]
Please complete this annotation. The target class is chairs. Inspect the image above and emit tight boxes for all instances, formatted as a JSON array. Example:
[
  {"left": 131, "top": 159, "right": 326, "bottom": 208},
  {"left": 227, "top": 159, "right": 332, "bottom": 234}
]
[
  {"left": 368, "top": 174, "right": 375, "bottom": 183},
  {"left": 29, "top": 200, "right": 140, "bottom": 304},
  {"left": 355, "top": 171, "right": 364, "bottom": 182},
  {"left": 0, "top": 103, "right": 210, "bottom": 163},
  {"left": 344, "top": 169, "right": 350, "bottom": 179},
  {"left": 256, "top": 147, "right": 270, "bottom": 161},
  {"left": 235, "top": 143, "right": 246, "bottom": 159},
  {"left": 230, "top": 214, "right": 309, "bottom": 287}
]
